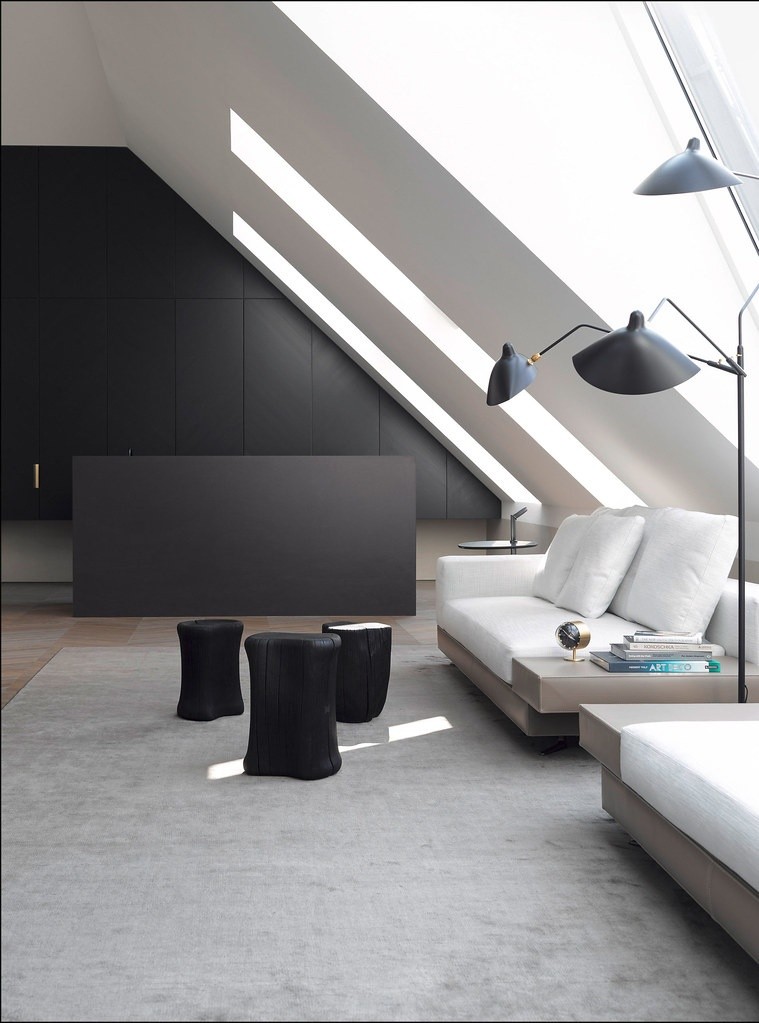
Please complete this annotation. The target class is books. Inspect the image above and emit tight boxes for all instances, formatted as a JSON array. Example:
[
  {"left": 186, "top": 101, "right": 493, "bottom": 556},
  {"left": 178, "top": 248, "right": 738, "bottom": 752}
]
[{"left": 589, "top": 631, "right": 720, "bottom": 673}]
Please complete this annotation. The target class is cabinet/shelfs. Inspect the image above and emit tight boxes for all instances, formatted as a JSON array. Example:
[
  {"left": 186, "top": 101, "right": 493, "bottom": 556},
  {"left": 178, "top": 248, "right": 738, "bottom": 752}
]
[{"left": 0, "top": 145, "right": 501, "bottom": 519}]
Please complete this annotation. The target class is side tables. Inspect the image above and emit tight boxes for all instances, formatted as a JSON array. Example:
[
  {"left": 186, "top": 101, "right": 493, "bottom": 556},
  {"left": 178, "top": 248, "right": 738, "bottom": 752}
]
[{"left": 457, "top": 540, "right": 538, "bottom": 555}]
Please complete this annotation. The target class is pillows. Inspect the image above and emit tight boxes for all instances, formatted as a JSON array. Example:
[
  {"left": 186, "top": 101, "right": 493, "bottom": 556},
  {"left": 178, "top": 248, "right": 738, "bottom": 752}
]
[
  {"left": 623, "top": 506, "right": 738, "bottom": 637},
  {"left": 532, "top": 514, "right": 597, "bottom": 605},
  {"left": 593, "top": 504, "right": 670, "bottom": 622},
  {"left": 554, "top": 514, "right": 647, "bottom": 619}
]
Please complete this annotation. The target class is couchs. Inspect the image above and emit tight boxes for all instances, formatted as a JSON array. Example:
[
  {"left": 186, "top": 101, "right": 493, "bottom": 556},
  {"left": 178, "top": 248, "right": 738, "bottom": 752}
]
[
  {"left": 578, "top": 703, "right": 759, "bottom": 966},
  {"left": 435, "top": 555, "right": 759, "bottom": 756}
]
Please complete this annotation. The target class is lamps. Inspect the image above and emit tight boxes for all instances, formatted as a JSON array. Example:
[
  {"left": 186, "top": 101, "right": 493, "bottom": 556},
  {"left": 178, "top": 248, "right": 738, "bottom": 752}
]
[{"left": 485, "top": 136, "right": 759, "bottom": 703}]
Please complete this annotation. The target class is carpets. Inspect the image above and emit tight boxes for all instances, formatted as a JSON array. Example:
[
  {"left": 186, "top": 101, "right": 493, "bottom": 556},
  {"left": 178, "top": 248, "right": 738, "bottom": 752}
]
[{"left": 1, "top": 647, "right": 759, "bottom": 1023}]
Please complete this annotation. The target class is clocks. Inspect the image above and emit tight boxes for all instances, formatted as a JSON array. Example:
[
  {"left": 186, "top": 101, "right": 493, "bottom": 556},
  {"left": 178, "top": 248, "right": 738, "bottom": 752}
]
[{"left": 556, "top": 621, "right": 591, "bottom": 662}]
